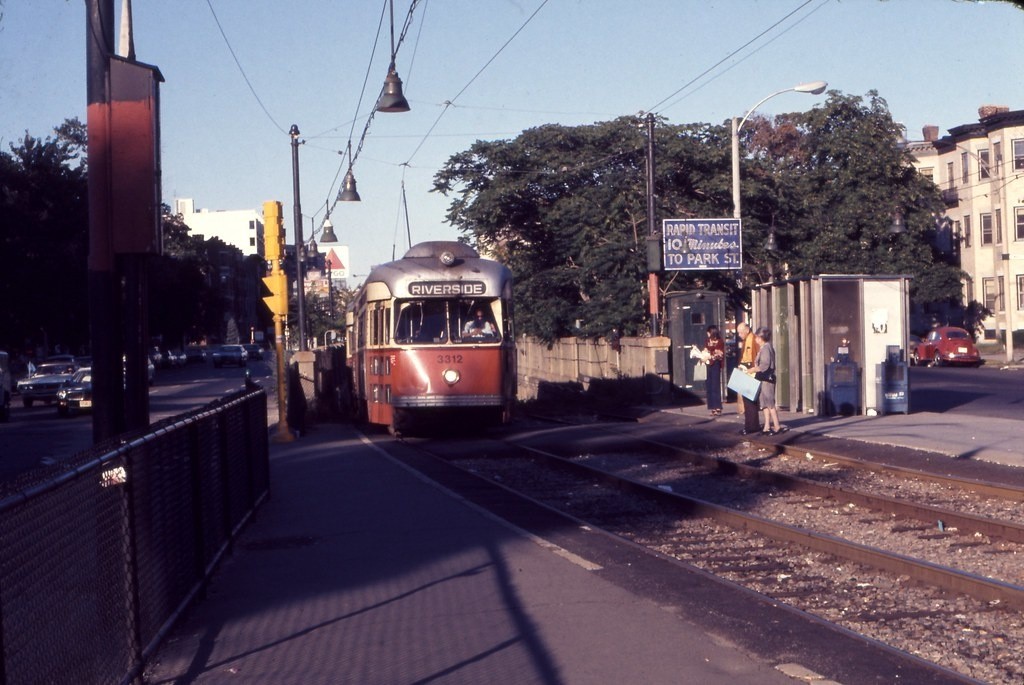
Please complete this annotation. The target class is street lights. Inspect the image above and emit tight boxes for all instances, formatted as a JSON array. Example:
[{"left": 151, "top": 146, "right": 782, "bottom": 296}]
[{"left": 735, "top": 81, "right": 830, "bottom": 415}]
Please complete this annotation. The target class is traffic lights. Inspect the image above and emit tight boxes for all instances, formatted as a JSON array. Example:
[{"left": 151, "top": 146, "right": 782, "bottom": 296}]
[{"left": 263, "top": 274, "right": 288, "bottom": 313}]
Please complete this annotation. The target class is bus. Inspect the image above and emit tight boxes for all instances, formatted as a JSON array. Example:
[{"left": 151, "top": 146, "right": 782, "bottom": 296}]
[{"left": 345, "top": 240, "right": 517, "bottom": 437}]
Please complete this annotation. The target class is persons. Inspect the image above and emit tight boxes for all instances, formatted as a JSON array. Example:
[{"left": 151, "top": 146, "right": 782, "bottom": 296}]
[
  {"left": 462, "top": 309, "right": 494, "bottom": 339},
  {"left": 736, "top": 323, "right": 762, "bottom": 434},
  {"left": 701, "top": 325, "right": 724, "bottom": 417},
  {"left": 745, "top": 328, "right": 782, "bottom": 436}
]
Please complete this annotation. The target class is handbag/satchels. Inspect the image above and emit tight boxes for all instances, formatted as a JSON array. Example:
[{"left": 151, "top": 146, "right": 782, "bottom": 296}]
[
  {"left": 694, "top": 361, "right": 707, "bottom": 380},
  {"left": 727, "top": 368, "right": 762, "bottom": 402},
  {"left": 754, "top": 345, "right": 777, "bottom": 384}
]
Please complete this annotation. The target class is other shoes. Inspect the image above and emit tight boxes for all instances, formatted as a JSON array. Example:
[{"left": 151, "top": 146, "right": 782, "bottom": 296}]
[
  {"left": 770, "top": 427, "right": 781, "bottom": 436},
  {"left": 738, "top": 427, "right": 760, "bottom": 435},
  {"left": 756, "top": 429, "right": 772, "bottom": 437},
  {"left": 710, "top": 411, "right": 715, "bottom": 416},
  {"left": 713, "top": 410, "right": 721, "bottom": 416}
]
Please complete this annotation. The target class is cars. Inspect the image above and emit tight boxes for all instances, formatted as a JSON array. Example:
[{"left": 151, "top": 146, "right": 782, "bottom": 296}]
[
  {"left": 725, "top": 336, "right": 743, "bottom": 357},
  {"left": 47, "top": 342, "right": 211, "bottom": 387},
  {"left": 56, "top": 368, "right": 94, "bottom": 413},
  {"left": 242, "top": 344, "right": 264, "bottom": 360},
  {"left": 18, "top": 362, "right": 80, "bottom": 410},
  {"left": 213, "top": 344, "right": 249, "bottom": 368},
  {"left": 914, "top": 327, "right": 980, "bottom": 369},
  {"left": 0, "top": 351, "right": 13, "bottom": 416}
]
